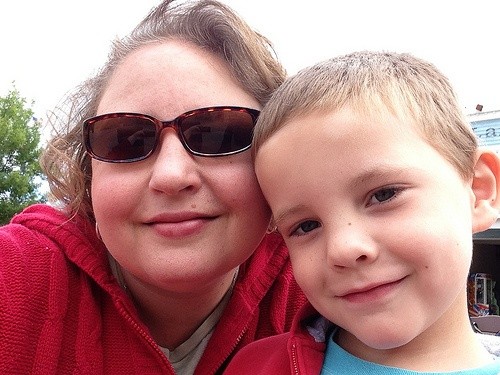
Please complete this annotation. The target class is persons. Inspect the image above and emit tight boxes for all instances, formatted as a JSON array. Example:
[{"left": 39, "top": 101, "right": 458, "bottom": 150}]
[
  {"left": 108, "top": 128, "right": 151, "bottom": 160},
  {"left": 221, "top": 51, "right": 500, "bottom": 375},
  {"left": 182, "top": 124, "right": 203, "bottom": 142},
  {"left": 218, "top": 116, "right": 254, "bottom": 153},
  {"left": 0, "top": 0, "right": 307, "bottom": 375}
]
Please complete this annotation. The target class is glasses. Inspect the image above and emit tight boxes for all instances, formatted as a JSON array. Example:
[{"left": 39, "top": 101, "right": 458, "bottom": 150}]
[{"left": 82, "top": 106, "right": 261, "bottom": 163}]
